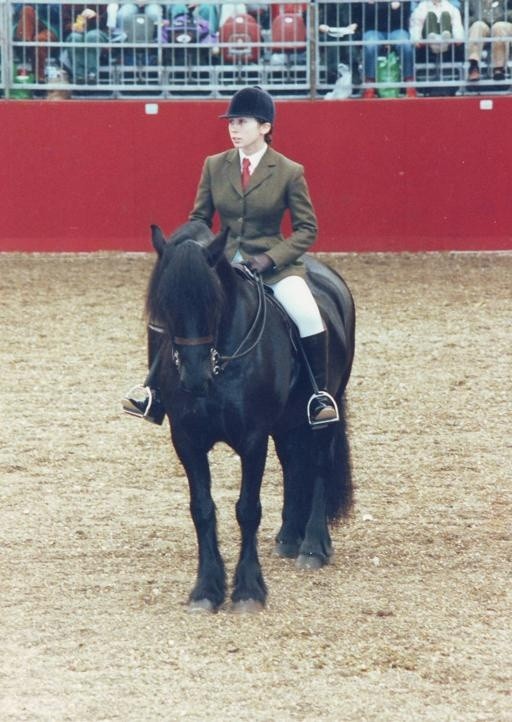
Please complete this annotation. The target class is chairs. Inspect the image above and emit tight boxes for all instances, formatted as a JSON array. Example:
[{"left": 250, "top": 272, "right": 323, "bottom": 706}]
[{"left": 111, "top": 12, "right": 312, "bottom": 93}]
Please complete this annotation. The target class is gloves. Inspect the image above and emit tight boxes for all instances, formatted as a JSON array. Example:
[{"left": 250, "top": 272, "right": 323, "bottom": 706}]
[{"left": 242, "top": 252, "right": 272, "bottom": 273}]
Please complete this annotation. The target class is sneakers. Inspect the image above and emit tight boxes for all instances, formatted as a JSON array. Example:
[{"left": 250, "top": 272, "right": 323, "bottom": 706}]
[
  {"left": 493, "top": 70, "right": 505, "bottom": 79},
  {"left": 468, "top": 67, "right": 480, "bottom": 80},
  {"left": 427, "top": 31, "right": 450, "bottom": 54},
  {"left": 363, "top": 89, "right": 375, "bottom": 98},
  {"left": 406, "top": 88, "right": 416, "bottom": 97}
]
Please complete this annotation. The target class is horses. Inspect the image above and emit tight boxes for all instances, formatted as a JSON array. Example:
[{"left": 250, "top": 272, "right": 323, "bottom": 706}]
[{"left": 137, "top": 222, "right": 357, "bottom": 616}]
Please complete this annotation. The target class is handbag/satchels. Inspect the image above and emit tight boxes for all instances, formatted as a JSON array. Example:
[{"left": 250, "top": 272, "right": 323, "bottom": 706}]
[{"left": 377, "top": 54, "right": 401, "bottom": 99}]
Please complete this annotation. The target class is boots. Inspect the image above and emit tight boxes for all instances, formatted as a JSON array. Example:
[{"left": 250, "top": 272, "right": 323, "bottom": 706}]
[
  {"left": 120, "top": 325, "right": 167, "bottom": 425},
  {"left": 301, "top": 331, "right": 337, "bottom": 431}
]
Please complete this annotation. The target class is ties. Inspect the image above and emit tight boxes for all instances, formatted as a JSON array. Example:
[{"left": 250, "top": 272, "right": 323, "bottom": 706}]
[{"left": 241, "top": 159, "right": 251, "bottom": 189}]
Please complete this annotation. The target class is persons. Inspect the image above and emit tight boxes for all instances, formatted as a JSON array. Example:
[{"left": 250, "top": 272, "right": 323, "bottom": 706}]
[{"left": 120, "top": 87, "right": 341, "bottom": 431}]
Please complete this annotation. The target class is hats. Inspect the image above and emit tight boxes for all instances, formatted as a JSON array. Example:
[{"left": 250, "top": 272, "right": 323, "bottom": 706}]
[{"left": 217, "top": 86, "right": 274, "bottom": 122}]
